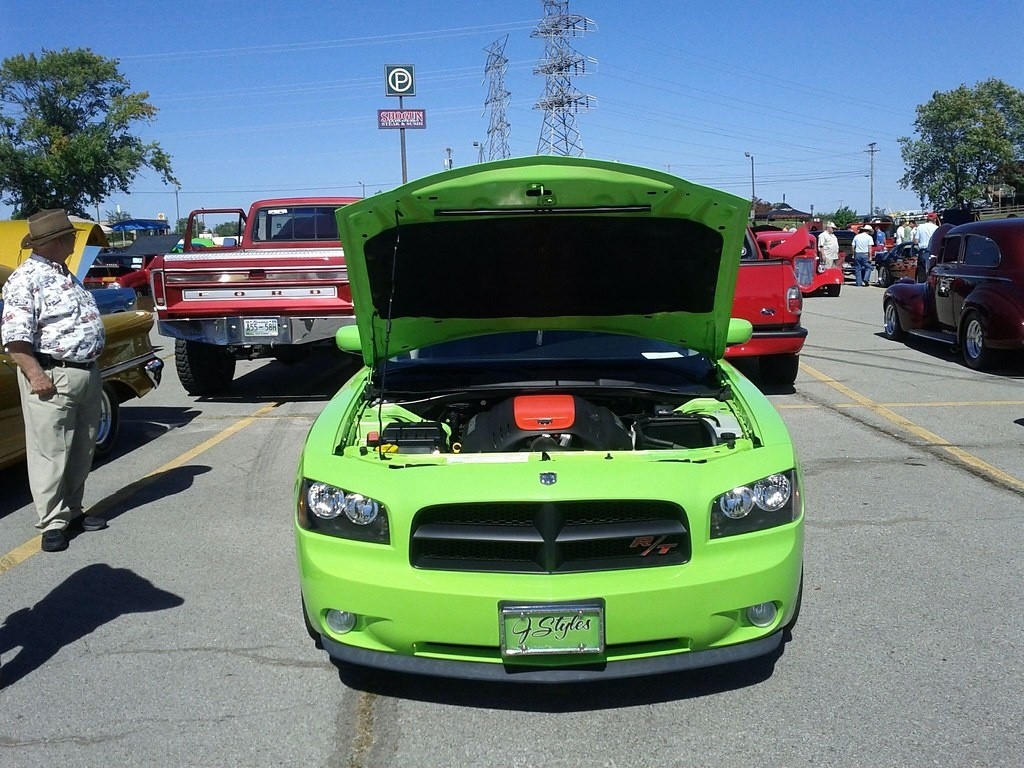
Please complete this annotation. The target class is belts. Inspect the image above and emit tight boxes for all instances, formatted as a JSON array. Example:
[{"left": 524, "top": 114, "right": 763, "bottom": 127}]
[{"left": 39, "top": 356, "right": 95, "bottom": 370}]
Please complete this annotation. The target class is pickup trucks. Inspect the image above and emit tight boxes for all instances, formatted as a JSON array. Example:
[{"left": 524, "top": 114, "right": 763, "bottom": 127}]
[
  {"left": 148, "top": 195, "right": 371, "bottom": 398},
  {"left": 721, "top": 224, "right": 807, "bottom": 387}
]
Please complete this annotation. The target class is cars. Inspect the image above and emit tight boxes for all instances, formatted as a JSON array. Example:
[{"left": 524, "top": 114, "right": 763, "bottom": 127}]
[
  {"left": 882, "top": 215, "right": 1024, "bottom": 372},
  {"left": 757, "top": 215, "right": 922, "bottom": 298},
  {"left": 0, "top": 218, "right": 184, "bottom": 478},
  {"left": 293, "top": 153, "right": 807, "bottom": 690}
]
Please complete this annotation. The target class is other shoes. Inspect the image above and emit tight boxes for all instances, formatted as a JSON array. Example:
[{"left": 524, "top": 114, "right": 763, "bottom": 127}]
[
  {"left": 41, "top": 529, "right": 69, "bottom": 551},
  {"left": 70, "top": 514, "right": 106, "bottom": 531},
  {"left": 862, "top": 280, "right": 869, "bottom": 286}
]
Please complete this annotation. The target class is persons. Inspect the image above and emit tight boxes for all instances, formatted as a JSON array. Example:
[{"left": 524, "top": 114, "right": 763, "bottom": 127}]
[
  {"left": 0, "top": 209, "right": 107, "bottom": 552},
  {"left": 780, "top": 212, "right": 939, "bottom": 287}
]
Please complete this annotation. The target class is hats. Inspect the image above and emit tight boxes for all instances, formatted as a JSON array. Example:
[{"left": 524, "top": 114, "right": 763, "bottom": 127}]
[
  {"left": 928, "top": 213, "right": 937, "bottom": 218},
  {"left": 860, "top": 225, "right": 874, "bottom": 235},
  {"left": 825, "top": 222, "right": 837, "bottom": 229},
  {"left": 21, "top": 207, "right": 87, "bottom": 249}
]
